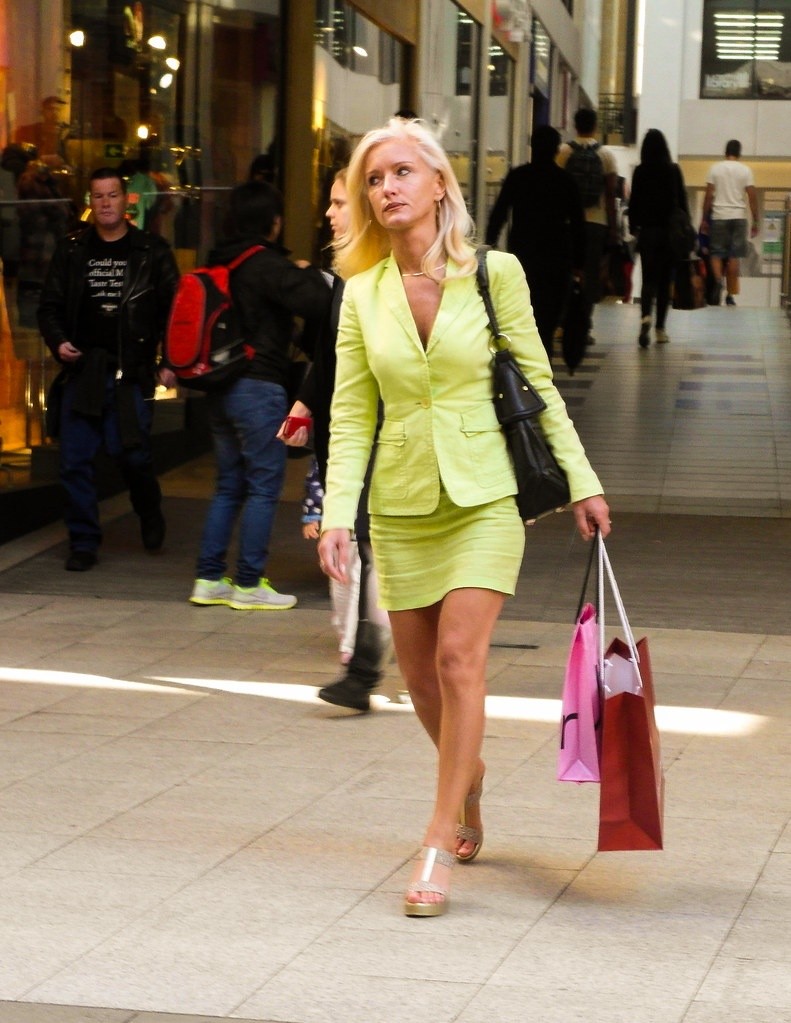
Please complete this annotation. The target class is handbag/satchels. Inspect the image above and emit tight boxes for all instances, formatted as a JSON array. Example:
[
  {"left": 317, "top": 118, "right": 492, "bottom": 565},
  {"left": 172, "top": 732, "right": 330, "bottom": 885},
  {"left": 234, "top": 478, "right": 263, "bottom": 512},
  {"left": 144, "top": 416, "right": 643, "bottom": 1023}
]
[
  {"left": 44, "top": 367, "right": 72, "bottom": 445},
  {"left": 490, "top": 349, "right": 572, "bottom": 521},
  {"left": 672, "top": 251, "right": 706, "bottom": 311},
  {"left": 555, "top": 601, "right": 668, "bottom": 851}
]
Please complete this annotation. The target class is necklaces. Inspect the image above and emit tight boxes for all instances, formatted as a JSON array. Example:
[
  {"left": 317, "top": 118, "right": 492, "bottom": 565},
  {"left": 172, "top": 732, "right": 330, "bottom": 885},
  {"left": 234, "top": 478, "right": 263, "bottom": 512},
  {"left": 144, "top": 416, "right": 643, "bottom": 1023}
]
[{"left": 402, "top": 263, "right": 446, "bottom": 276}]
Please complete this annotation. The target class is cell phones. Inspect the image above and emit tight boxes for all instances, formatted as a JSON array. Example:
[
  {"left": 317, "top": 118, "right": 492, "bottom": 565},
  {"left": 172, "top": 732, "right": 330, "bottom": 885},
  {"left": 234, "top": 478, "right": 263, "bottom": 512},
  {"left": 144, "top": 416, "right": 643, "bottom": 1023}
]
[{"left": 283, "top": 416, "right": 314, "bottom": 437}]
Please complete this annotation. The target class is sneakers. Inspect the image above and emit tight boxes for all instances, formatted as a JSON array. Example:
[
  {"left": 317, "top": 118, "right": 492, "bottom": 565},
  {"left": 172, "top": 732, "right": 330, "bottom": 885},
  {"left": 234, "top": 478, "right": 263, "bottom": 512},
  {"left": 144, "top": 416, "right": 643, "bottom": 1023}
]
[
  {"left": 189, "top": 576, "right": 234, "bottom": 606},
  {"left": 232, "top": 579, "right": 298, "bottom": 612}
]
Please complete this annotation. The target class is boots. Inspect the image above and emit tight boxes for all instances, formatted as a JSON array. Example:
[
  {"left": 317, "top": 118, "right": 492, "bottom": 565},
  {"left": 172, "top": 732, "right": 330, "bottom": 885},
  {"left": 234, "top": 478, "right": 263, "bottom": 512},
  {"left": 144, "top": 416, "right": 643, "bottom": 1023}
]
[{"left": 318, "top": 619, "right": 393, "bottom": 713}]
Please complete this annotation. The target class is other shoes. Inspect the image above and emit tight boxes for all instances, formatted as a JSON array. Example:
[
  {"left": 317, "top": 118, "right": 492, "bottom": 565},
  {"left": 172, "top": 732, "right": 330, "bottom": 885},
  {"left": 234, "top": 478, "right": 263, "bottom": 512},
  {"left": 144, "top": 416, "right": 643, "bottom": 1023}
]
[
  {"left": 140, "top": 537, "right": 164, "bottom": 555},
  {"left": 637, "top": 315, "right": 651, "bottom": 347},
  {"left": 707, "top": 282, "right": 721, "bottom": 305},
  {"left": 65, "top": 554, "right": 100, "bottom": 571},
  {"left": 655, "top": 329, "right": 669, "bottom": 344},
  {"left": 726, "top": 293, "right": 736, "bottom": 305}
]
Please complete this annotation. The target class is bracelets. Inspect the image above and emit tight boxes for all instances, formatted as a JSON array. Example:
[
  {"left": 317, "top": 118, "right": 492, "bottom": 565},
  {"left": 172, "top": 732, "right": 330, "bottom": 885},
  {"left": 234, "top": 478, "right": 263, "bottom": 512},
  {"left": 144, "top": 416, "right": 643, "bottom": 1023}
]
[{"left": 751, "top": 220, "right": 760, "bottom": 226}]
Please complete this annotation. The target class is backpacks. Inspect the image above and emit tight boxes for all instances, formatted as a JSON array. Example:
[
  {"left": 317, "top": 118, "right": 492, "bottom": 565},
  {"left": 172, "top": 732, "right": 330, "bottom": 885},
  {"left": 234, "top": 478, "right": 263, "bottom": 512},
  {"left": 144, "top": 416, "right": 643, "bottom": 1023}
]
[
  {"left": 161, "top": 246, "right": 264, "bottom": 393},
  {"left": 566, "top": 141, "right": 607, "bottom": 208}
]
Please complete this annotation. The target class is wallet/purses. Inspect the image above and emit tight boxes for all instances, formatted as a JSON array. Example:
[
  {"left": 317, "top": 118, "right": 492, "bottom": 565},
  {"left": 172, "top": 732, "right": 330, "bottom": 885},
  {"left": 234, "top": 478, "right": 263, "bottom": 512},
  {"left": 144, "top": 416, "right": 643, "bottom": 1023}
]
[{"left": 282, "top": 416, "right": 314, "bottom": 439}]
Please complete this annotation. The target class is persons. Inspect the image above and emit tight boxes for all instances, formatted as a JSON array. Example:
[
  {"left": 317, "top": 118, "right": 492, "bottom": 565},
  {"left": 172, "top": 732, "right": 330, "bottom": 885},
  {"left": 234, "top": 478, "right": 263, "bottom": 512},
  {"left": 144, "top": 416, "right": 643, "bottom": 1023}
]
[
  {"left": 485, "top": 125, "right": 569, "bottom": 363},
  {"left": 699, "top": 139, "right": 762, "bottom": 305},
  {"left": 319, "top": 112, "right": 612, "bottom": 916},
  {"left": 38, "top": 167, "right": 181, "bottom": 571},
  {"left": 184, "top": 157, "right": 415, "bottom": 710},
  {"left": 555, "top": 108, "right": 616, "bottom": 342},
  {"left": 0, "top": 97, "right": 82, "bottom": 281},
  {"left": 630, "top": 128, "right": 692, "bottom": 346}
]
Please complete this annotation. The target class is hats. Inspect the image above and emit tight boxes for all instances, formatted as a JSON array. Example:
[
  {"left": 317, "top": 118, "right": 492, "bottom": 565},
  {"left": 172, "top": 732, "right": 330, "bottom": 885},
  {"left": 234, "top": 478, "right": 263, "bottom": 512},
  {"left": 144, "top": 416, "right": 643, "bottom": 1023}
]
[{"left": 43, "top": 96, "right": 67, "bottom": 105}]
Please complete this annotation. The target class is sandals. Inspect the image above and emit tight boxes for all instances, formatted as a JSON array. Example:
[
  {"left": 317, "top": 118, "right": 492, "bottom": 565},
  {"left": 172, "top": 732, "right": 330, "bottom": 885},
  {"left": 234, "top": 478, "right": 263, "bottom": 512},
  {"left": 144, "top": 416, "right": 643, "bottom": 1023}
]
[
  {"left": 403, "top": 847, "right": 452, "bottom": 918},
  {"left": 453, "top": 757, "right": 485, "bottom": 864}
]
